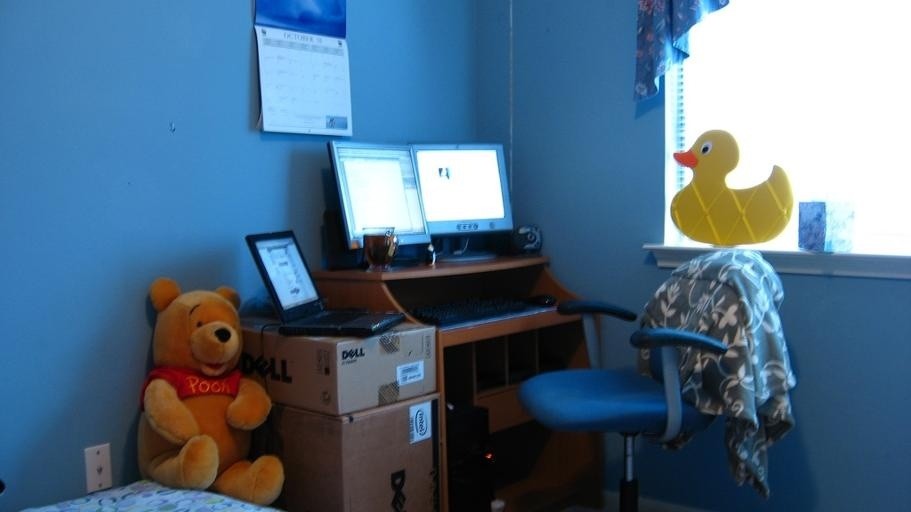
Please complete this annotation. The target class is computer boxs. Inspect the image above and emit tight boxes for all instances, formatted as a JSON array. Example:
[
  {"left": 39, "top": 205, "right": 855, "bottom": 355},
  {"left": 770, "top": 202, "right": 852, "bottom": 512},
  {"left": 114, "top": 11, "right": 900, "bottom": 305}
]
[{"left": 445, "top": 405, "right": 496, "bottom": 512}]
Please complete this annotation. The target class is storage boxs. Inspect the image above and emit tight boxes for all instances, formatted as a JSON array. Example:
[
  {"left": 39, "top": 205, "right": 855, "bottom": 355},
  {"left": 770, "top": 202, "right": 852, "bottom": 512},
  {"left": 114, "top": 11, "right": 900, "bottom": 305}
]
[
  {"left": 239, "top": 319, "right": 436, "bottom": 416},
  {"left": 249, "top": 400, "right": 434, "bottom": 512}
]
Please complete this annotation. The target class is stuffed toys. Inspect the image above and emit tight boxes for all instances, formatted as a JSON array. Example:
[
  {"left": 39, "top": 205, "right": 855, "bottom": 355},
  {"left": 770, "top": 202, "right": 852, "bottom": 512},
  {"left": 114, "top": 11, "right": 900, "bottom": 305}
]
[{"left": 134, "top": 277, "right": 287, "bottom": 507}]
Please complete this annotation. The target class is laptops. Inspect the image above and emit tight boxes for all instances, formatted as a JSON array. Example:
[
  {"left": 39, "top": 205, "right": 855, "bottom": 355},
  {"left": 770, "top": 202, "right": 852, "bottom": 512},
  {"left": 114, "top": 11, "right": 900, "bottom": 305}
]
[{"left": 246, "top": 230, "right": 406, "bottom": 337}]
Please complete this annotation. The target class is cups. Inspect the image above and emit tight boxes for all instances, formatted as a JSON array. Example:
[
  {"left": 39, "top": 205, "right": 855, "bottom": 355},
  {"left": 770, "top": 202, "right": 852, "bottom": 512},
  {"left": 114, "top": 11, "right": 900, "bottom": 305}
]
[{"left": 361, "top": 225, "right": 398, "bottom": 273}]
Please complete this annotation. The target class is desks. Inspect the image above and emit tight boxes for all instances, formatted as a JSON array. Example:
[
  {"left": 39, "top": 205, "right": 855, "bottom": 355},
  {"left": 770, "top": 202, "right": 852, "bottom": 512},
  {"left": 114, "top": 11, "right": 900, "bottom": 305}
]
[{"left": 311, "top": 255, "right": 605, "bottom": 512}]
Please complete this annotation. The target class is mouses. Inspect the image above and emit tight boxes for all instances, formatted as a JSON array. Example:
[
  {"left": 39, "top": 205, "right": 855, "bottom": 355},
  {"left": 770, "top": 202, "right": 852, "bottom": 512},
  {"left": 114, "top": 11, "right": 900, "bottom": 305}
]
[{"left": 533, "top": 295, "right": 556, "bottom": 306}]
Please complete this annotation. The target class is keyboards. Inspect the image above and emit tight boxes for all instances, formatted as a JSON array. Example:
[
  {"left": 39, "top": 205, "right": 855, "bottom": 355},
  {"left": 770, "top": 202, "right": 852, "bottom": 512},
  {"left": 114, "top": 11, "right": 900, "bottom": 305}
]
[{"left": 409, "top": 295, "right": 529, "bottom": 327}]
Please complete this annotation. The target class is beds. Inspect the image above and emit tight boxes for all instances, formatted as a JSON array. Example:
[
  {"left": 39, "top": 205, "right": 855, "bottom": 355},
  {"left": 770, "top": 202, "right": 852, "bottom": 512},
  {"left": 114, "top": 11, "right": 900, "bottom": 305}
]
[{"left": 19, "top": 480, "right": 286, "bottom": 512}]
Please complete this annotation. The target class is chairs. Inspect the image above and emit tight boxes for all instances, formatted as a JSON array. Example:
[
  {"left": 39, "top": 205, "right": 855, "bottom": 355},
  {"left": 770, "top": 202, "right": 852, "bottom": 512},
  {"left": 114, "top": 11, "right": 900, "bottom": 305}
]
[{"left": 519, "top": 250, "right": 762, "bottom": 512}]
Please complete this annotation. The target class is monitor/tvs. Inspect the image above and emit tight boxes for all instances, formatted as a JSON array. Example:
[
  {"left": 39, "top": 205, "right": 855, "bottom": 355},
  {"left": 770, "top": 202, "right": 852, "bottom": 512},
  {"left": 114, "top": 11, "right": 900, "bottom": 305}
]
[
  {"left": 327, "top": 140, "right": 432, "bottom": 271},
  {"left": 408, "top": 143, "right": 514, "bottom": 263}
]
